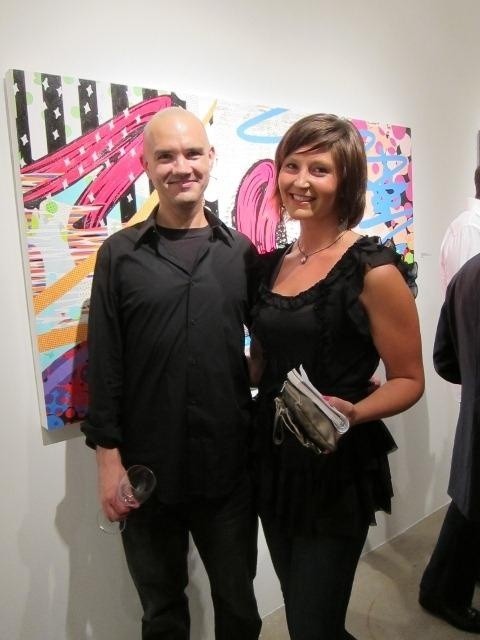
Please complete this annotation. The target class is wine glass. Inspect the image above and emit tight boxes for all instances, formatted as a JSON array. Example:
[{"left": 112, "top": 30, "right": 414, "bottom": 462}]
[{"left": 96, "top": 462, "right": 158, "bottom": 536}]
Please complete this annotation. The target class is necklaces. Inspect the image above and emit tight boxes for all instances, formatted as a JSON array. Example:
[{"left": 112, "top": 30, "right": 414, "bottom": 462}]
[{"left": 298, "top": 228, "right": 350, "bottom": 265}]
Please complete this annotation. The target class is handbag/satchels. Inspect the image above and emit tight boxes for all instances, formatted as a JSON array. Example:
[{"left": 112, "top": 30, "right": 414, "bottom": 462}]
[{"left": 272, "top": 380, "right": 337, "bottom": 454}]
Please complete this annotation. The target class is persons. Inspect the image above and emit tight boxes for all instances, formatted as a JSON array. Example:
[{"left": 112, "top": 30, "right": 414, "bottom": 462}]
[
  {"left": 437, "top": 164, "right": 480, "bottom": 290},
  {"left": 413, "top": 252, "right": 480, "bottom": 634},
  {"left": 240, "top": 111, "right": 427, "bottom": 640},
  {"left": 79, "top": 103, "right": 384, "bottom": 640}
]
